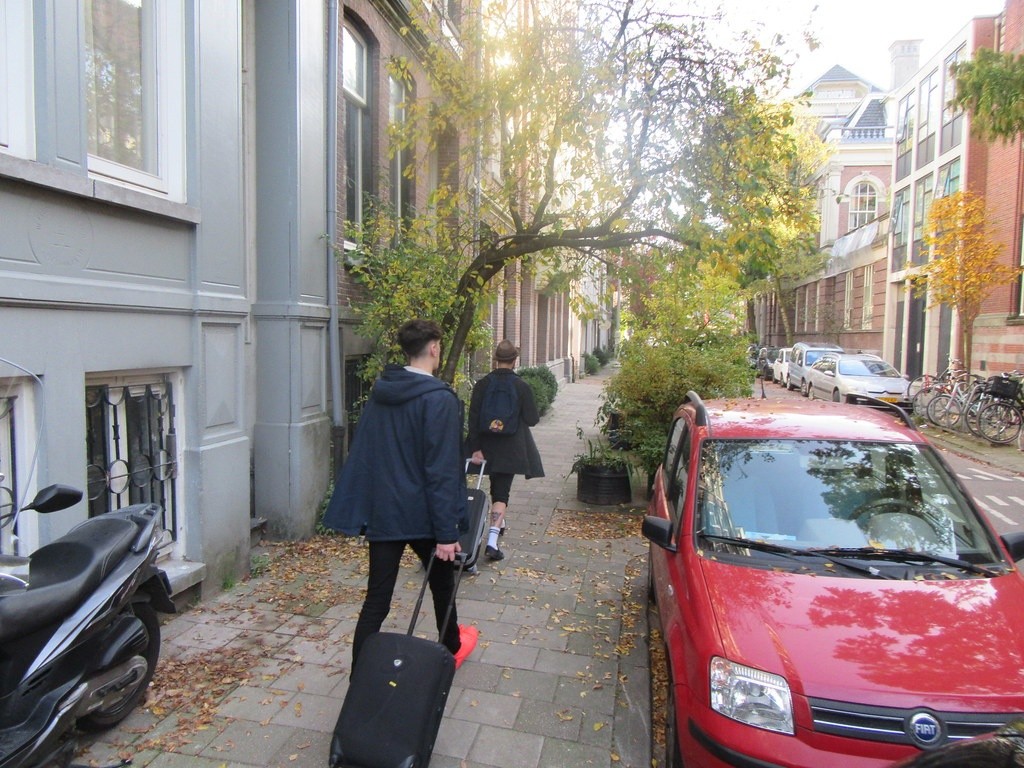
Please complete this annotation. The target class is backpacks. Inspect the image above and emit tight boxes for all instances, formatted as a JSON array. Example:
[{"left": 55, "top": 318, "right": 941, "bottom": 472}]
[{"left": 478, "top": 372, "right": 522, "bottom": 437}]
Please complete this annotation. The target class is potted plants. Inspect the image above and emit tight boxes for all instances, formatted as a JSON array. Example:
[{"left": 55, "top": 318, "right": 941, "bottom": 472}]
[{"left": 564, "top": 340, "right": 759, "bottom": 507}]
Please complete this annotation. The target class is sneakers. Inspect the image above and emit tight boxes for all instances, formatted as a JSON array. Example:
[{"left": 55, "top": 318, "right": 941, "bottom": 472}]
[
  {"left": 453, "top": 620, "right": 479, "bottom": 671},
  {"left": 485, "top": 545, "right": 505, "bottom": 560},
  {"left": 500, "top": 520, "right": 506, "bottom": 536}
]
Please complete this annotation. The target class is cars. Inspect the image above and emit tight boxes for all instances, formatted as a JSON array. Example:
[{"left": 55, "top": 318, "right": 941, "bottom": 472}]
[
  {"left": 640, "top": 392, "right": 1024, "bottom": 767},
  {"left": 805, "top": 352, "right": 916, "bottom": 420},
  {"left": 745, "top": 344, "right": 769, "bottom": 368},
  {"left": 772, "top": 348, "right": 793, "bottom": 387},
  {"left": 755, "top": 345, "right": 787, "bottom": 380}
]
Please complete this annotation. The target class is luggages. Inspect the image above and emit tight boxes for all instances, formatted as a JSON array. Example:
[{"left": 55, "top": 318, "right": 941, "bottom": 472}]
[
  {"left": 328, "top": 548, "right": 468, "bottom": 768},
  {"left": 454, "top": 457, "right": 488, "bottom": 574}
]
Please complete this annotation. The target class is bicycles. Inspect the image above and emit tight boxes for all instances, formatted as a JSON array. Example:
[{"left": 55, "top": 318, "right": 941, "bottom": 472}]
[{"left": 906, "top": 353, "right": 1024, "bottom": 444}]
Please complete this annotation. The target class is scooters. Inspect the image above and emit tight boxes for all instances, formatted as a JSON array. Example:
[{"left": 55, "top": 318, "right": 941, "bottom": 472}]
[{"left": 0, "top": 482, "right": 177, "bottom": 768}]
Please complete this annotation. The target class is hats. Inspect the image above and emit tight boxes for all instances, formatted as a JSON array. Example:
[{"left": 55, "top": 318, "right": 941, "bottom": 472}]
[{"left": 492, "top": 339, "right": 522, "bottom": 361}]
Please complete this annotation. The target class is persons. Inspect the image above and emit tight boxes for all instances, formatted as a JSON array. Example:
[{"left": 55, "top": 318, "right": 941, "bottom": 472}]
[
  {"left": 321, "top": 321, "right": 477, "bottom": 684},
  {"left": 464, "top": 341, "right": 545, "bottom": 560}
]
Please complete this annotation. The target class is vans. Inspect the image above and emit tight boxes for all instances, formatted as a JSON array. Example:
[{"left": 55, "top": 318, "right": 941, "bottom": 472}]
[{"left": 786, "top": 341, "right": 845, "bottom": 397}]
